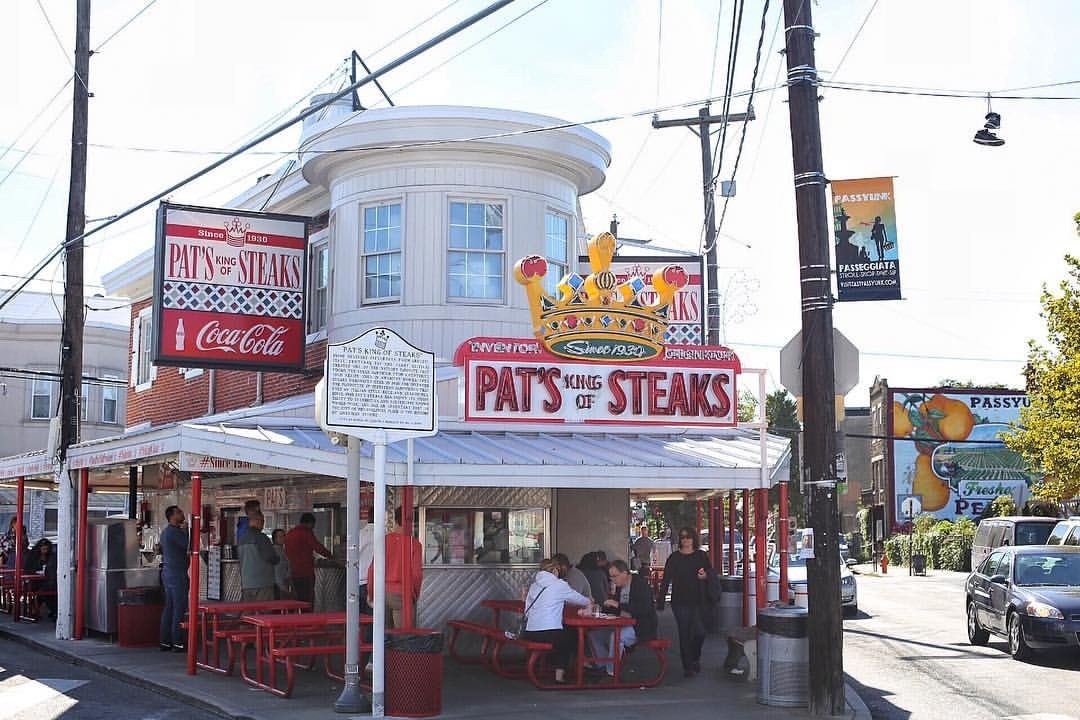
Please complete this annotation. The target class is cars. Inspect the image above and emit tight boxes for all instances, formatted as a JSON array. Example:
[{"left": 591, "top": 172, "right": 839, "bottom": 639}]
[
  {"left": 964, "top": 544, "right": 1080, "bottom": 661},
  {"left": 701, "top": 527, "right": 746, "bottom": 567},
  {"left": 749, "top": 527, "right": 859, "bottom": 617},
  {"left": 1045, "top": 516, "right": 1080, "bottom": 547}
]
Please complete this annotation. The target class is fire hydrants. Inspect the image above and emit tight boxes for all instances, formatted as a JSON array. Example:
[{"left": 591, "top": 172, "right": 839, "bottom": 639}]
[{"left": 878, "top": 552, "right": 890, "bottom": 574}]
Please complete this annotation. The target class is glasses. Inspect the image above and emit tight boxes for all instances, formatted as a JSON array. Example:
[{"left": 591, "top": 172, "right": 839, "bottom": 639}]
[
  {"left": 609, "top": 571, "right": 622, "bottom": 579},
  {"left": 679, "top": 534, "right": 692, "bottom": 539}
]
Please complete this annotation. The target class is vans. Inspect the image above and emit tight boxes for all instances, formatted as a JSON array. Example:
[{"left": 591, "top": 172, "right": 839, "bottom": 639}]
[{"left": 970, "top": 515, "right": 1067, "bottom": 573}]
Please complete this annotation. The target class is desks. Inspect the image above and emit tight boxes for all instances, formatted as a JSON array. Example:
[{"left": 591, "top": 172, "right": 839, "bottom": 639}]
[
  {"left": 644, "top": 575, "right": 664, "bottom": 600},
  {"left": 197, "top": 600, "right": 312, "bottom": 670},
  {"left": 14, "top": 575, "right": 48, "bottom": 624},
  {"left": 241, "top": 611, "right": 373, "bottom": 693},
  {"left": 0, "top": 564, "right": 26, "bottom": 614},
  {"left": 480, "top": 599, "right": 636, "bottom": 690}
]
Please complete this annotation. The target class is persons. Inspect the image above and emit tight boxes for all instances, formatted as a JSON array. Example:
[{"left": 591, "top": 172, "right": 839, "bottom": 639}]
[
  {"left": 576, "top": 551, "right": 612, "bottom": 607},
  {"left": 285, "top": 513, "right": 333, "bottom": 613},
  {"left": 553, "top": 553, "right": 592, "bottom": 598},
  {"left": 239, "top": 511, "right": 281, "bottom": 631},
  {"left": 585, "top": 559, "right": 653, "bottom": 681},
  {"left": 0, "top": 516, "right": 57, "bottom": 620},
  {"left": 237, "top": 500, "right": 260, "bottom": 544},
  {"left": 656, "top": 527, "right": 714, "bottom": 677},
  {"left": 159, "top": 506, "right": 189, "bottom": 652},
  {"left": 523, "top": 559, "right": 590, "bottom": 684},
  {"left": 357, "top": 504, "right": 423, "bottom": 670},
  {"left": 633, "top": 527, "right": 656, "bottom": 568},
  {"left": 271, "top": 529, "right": 286, "bottom": 600}
]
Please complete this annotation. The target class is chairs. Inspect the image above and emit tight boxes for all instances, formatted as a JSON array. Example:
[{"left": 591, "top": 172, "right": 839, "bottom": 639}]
[{"left": 1022, "top": 564, "right": 1072, "bottom": 583}]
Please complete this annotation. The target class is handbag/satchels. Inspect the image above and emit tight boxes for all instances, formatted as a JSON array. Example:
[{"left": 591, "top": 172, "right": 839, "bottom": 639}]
[{"left": 518, "top": 616, "right": 528, "bottom": 639}]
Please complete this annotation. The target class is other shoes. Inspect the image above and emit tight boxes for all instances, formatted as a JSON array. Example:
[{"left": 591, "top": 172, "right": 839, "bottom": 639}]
[
  {"left": 684, "top": 670, "right": 693, "bottom": 676},
  {"left": 172, "top": 643, "right": 187, "bottom": 653},
  {"left": 692, "top": 661, "right": 700, "bottom": 673},
  {"left": 585, "top": 664, "right": 614, "bottom": 682},
  {"left": 160, "top": 642, "right": 173, "bottom": 651}
]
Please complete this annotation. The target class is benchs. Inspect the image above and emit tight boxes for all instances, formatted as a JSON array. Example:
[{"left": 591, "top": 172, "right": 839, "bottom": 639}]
[
  {"left": 445, "top": 619, "right": 553, "bottom": 690},
  {"left": 28, "top": 591, "right": 57, "bottom": 621},
  {"left": 625, "top": 638, "right": 671, "bottom": 687},
  {"left": 180, "top": 620, "right": 375, "bottom": 697},
  {"left": 0, "top": 579, "right": 30, "bottom": 610}
]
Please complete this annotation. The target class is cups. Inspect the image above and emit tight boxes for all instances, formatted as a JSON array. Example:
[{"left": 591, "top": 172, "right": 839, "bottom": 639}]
[
  {"left": 521, "top": 583, "right": 529, "bottom": 602},
  {"left": 224, "top": 544, "right": 233, "bottom": 559},
  {"left": 591, "top": 605, "right": 599, "bottom": 619}
]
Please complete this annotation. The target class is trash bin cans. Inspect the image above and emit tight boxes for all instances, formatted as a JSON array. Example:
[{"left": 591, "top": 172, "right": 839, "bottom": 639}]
[
  {"left": 116, "top": 586, "right": 165, "bottom": 648},
  {"left": 712, "top": 575, "right": 742, "bottom": 630},
  {"left": 757, "top": 607, "right": 810, "bottom": 707},
  {"left": 912, "top": 549, "right": 927, "bottom": 576},
  {"left": 384, "top": 629, "right": 443, "bottom": 716}
]
